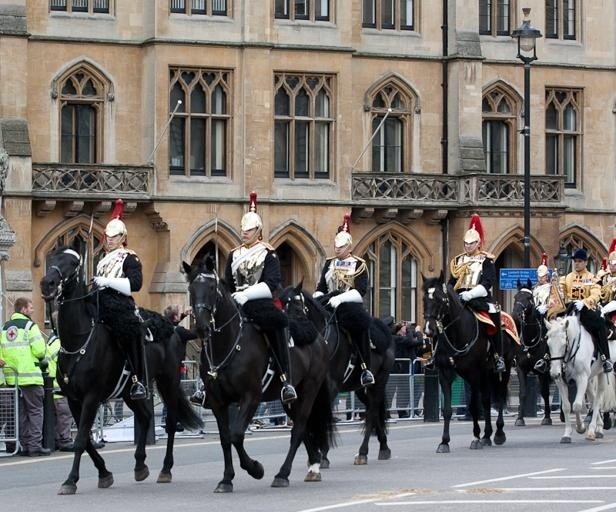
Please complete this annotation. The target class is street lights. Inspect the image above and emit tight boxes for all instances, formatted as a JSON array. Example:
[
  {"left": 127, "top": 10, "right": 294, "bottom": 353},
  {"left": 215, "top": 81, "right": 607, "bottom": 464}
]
[
  {"left": 553, "top": 240, "right": 571, "bottom": 276},
  {"left": 514, "top": 5, "right": 550, "bottom": 301}
]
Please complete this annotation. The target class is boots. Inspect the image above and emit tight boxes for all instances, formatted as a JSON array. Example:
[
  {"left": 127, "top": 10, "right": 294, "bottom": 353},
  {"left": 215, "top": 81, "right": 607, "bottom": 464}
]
[
  {"left": 267, "top": 326, "right": 297, "bottom": 403},
  {"left": 357, "top": 326, "right": 373, "bottom": 382},
  {"left": 122, "top": 333, "right": 147, "bottom": 398},
  {"left": 488, "top": 311, "right": 505, "bottom": 372}
]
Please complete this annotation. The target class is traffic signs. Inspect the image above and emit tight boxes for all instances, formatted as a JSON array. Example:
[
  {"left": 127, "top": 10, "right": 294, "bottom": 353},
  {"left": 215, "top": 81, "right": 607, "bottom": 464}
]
[{"left": 498, "top": 268, "right": 552, "bottom": 291}]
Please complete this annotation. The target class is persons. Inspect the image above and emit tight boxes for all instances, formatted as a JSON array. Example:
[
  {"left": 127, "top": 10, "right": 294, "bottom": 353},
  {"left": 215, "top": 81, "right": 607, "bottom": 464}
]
[
  {"left": 315, "top": 214, "right": 373, "bottom": 384},
  {"left": 162, "top": 305, "right": 197, "bottom": 431},
  {"left": 226, "top": 191, "right": 295, "bottom": 399},
  {"left": 514, "top": 239, "right": 616, "bottom": 410},
  {"left": 450, "top": 214, "right": 505, "bottom": 375},
  {"left": 0, "top": 297, "right": 105, "bottom": 456},
  {"left": 89, "top": 199, "right": 147, "bottom": 400},
  {"left": 251, "top": 317, "right": 492, "bottom": 429}
]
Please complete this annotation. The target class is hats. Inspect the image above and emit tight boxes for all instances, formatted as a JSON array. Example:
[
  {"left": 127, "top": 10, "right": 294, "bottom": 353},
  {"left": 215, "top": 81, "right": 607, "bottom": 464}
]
[
  {"left": 597, "top": 258, "right": 610, "bottom": 279},
  {"left": 241, "top": 191, "right": 262, "bottom": 231},
  {"left": 537, "top": 253, "right": 549, "bottom": 277},
  {"left": 381, "top": 316, "right": 394, "bottom": 324},
  {"left": 570, "top": 248, "right": 593, "bottom": 261},
  {"left": 608, "top": 239, "right": 615, "bottom": 265},
  {"left": 334, "top": 213, "right": 352, "bottom": 248},
  {"left": 464, "top": 213, "right": 484, "bottom": 244},
  {"left": 104, "top": 198, "right": 127, "bottom": 237}
]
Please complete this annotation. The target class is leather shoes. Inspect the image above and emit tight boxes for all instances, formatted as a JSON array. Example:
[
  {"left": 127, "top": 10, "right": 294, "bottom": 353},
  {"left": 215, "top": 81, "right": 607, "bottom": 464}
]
[
  {"left": 27, "top": 447, "right": 51, "bottom": 457},
  {"left": 60, "top": 445, "right": 73, "bottom": 452},
  {"left": 602, "top": 359, "right": 612, "bottom": 372},
  {"left": 92, "top": 442, "right": 104, "bottom": 449}
]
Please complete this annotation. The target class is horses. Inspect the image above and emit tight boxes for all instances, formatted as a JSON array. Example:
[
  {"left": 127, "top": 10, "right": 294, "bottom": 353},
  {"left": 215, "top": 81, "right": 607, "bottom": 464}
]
[
  {"left": 273, "top": 274, "right": 399, "bottom": 468},
  {"left": 37, "top": 232, "right": 204, "bottom": 496},
  {"left": 582, "top": 404, "right": 615, "bottom": 431},
  {"left": 510, "top": 276, "right": 569, "bottom": 428},
  {"left": 543, "top": 311, "right": 616, "bottom": 444},
  {"left": 180, "top": 254, "right": 338, "bottom": 495},
  {"left": 419, "top": 269, "right": 520, "bottom": 454}
]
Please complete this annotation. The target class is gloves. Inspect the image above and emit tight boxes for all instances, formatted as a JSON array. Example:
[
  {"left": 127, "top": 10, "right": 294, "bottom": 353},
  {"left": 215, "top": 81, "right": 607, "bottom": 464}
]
[
  {"left": 230, "top": 282, "right": 272, "bottom": 306},
  {"left": 328, "top": 288, "right": 362, "bottom": 308},
  {"left": 90, "top": 276, "right": 131, "bottom": 296},
  {"left": 458, "top": 284, "right": 487, "bottom": 302},
  {"left": 572, "top": 300, "right": 583, "bottom": 311},
  {"left": 600, "top": 301, "right": 616, "bottom": 318},
  {"left": 536, "top": 305, "right": 546, "bottom": 314},
  {"left": 312, "top": 291, "right": 324, "bottom": 298}
]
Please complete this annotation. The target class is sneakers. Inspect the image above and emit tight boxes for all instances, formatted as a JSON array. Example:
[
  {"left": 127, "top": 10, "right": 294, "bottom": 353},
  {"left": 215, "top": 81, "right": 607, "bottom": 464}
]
[
  {"left": 492, "top": 406, "right": 561, "bottom": 418},
  {"left": 248, "top": 420, "right": 266, "bottom": 428}
]
